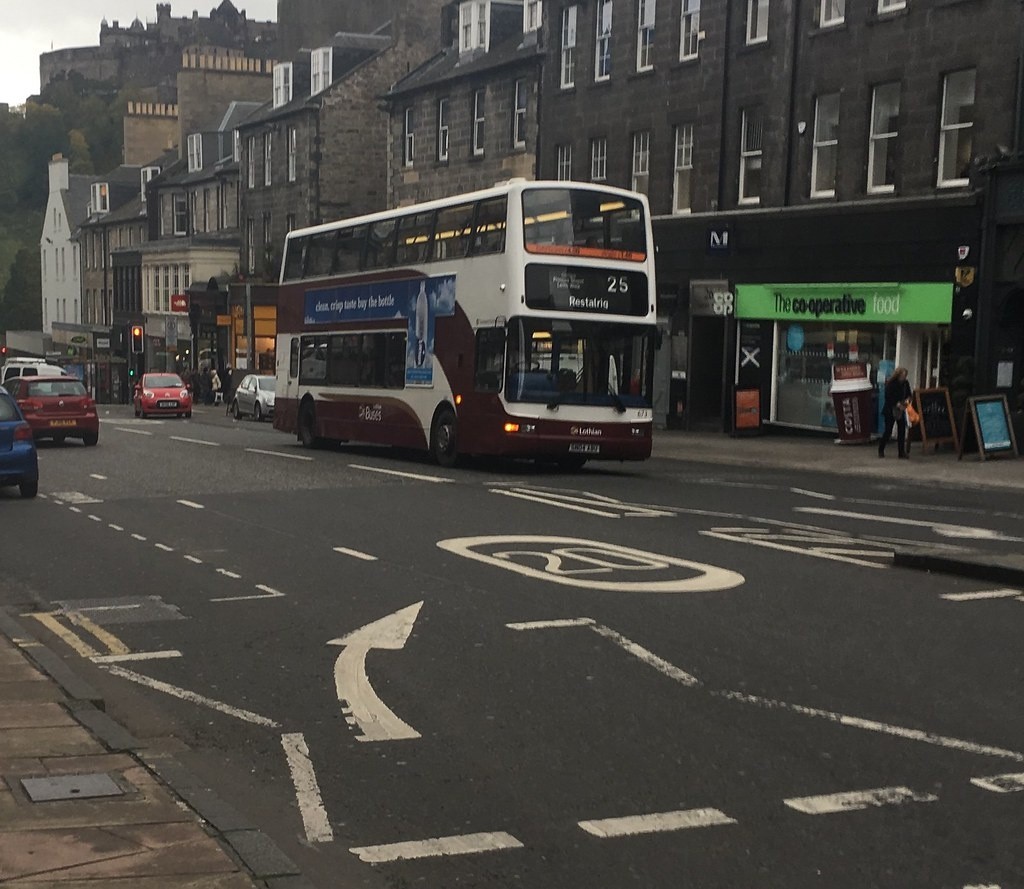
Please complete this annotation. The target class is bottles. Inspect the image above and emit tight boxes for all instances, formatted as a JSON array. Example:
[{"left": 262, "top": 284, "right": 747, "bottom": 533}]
[{"left": 413, "top": 280, "right": 428, "bottom": 385}]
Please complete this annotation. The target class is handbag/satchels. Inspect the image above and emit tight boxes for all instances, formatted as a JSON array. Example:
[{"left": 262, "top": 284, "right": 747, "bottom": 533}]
[
  {"left": 905, "top": 396, "right": 919, "bottom": 428},
  {"left": 214, "top": 390, "right": 224, "bottom": 403}
]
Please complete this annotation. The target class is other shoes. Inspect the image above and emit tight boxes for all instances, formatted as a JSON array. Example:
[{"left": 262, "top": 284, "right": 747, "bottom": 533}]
[
  {"left": 898, "top": 453, "right": 909, "bottom": 459},
  {"left": 878, "top": 447, "right": 884, "bottom": 458}
]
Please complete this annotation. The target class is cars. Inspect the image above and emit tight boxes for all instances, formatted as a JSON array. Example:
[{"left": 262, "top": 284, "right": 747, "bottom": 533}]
[
  {"left": 134, "top": 372, "right": 193, "bottom": 419},
  {"left": 0, "top": 386, "right": 39, "bottom": 497},
  {"left": 0, "top": 374, "right": 99, "bottom": 446},
  {"left": 232, "top": 374, "right": 278, "bottom": 421}
]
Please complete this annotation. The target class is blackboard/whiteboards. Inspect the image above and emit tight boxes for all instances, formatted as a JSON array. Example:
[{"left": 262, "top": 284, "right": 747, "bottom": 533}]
[{"left": 968, "top": 394, "right": 1018, "bottom": 458}]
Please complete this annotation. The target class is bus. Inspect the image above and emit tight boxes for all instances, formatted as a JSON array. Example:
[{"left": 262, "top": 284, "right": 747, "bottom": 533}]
[{"left": 275, "top": 174, "right": 664, "bottom": 467}]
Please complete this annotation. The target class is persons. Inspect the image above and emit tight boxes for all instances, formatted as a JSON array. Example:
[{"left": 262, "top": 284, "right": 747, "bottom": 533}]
[
  {"left": 180, "top": 366, "right": 221, "bottom": 407},
  {"left": 878, "top": 367, "right": 913, "bottom": 459}
]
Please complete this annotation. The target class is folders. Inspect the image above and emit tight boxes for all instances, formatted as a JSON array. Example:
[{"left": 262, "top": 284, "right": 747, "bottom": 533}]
[{"left": 915, "top": 387, "right": 958, "bottom": 445}]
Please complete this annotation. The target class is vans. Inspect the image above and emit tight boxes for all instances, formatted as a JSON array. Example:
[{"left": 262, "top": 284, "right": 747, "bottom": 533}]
[{"left": 0, "top": 356, "right": 75, "bottom": 392}]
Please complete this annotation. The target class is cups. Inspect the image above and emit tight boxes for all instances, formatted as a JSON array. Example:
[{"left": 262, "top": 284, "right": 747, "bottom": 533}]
[{"left": 830, "top": 378, "right": 873, "bottom": 440}]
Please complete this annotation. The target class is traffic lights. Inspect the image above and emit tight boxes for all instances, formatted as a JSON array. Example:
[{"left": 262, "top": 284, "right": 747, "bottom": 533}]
[
  {"left": 130, "top": 368, "right": 135, "bottom": 377},
  {"left": 131, "top": 327, "right": 144, "bottom": 355}
]
